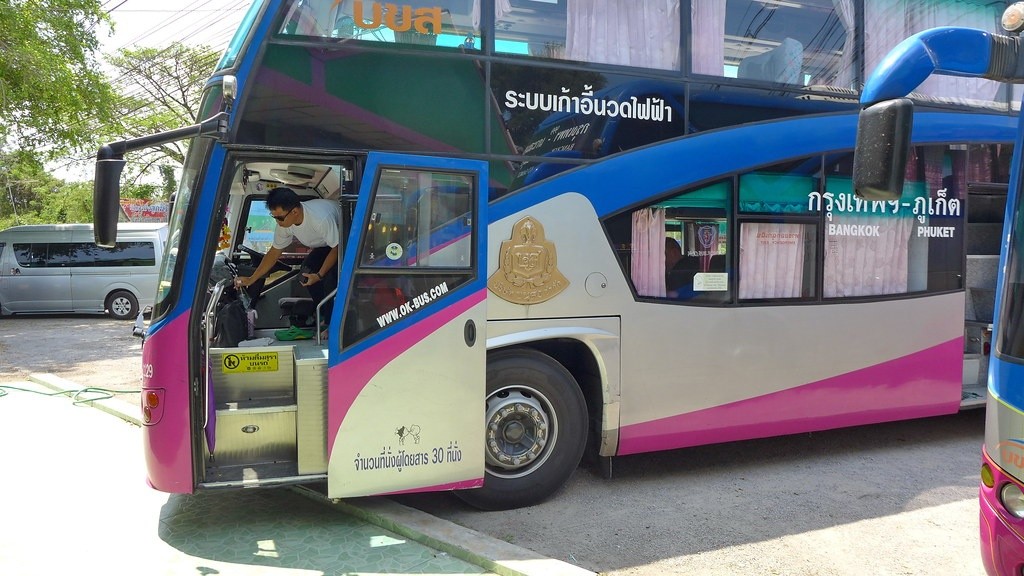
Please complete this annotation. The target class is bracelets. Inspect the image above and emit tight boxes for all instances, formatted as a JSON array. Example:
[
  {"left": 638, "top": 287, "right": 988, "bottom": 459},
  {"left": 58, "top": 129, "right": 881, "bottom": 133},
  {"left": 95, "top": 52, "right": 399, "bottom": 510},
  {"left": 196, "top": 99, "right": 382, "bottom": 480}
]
[{"left": 315, "top": 272, "right": 324, "bottom": 282}]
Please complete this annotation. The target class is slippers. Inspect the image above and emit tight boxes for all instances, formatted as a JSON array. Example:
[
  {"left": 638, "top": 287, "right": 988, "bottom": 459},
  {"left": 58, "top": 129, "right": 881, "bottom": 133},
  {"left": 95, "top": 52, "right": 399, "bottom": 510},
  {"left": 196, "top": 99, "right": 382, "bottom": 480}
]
[{"left": 274, "top": 326, "right": 315, "bottom": 341}]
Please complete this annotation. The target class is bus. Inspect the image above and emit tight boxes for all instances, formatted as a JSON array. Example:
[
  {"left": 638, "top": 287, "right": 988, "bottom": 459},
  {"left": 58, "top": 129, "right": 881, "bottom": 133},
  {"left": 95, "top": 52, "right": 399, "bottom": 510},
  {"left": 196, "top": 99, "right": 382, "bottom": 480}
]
[
  {"left": 849, "top": 1, "right": 1024, "bottom": 576},
  {"left": 92, "top": 0, "right": 987, "bottom": 511}
]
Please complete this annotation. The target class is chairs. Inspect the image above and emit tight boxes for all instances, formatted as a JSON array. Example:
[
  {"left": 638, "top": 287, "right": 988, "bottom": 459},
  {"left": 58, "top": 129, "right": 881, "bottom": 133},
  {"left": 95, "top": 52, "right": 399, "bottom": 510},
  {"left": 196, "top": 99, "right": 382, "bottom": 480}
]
[{"left": 685, "top": 252, "right": 728, "bottom": 276}]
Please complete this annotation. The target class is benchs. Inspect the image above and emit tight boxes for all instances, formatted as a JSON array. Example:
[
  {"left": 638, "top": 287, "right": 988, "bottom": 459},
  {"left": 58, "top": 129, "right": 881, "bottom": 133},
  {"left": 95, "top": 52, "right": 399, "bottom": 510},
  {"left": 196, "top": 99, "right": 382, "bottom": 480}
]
[{"left": 736, "top": 36, "right": 806, "bottom": 86}]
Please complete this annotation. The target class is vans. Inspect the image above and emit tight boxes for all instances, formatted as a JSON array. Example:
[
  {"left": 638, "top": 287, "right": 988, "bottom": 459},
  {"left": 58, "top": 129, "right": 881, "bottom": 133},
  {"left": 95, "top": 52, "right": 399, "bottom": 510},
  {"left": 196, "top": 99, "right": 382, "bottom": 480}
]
[{"left": 1, "top": 222, "right": 168, "bottom": 320}]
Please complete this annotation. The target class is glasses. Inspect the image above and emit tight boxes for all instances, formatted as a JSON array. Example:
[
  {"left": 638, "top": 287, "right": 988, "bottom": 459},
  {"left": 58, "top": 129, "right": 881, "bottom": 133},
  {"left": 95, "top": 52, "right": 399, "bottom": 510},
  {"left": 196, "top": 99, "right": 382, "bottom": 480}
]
[{"left": 270, "top": 206, "right": 298, "bottom": 221}]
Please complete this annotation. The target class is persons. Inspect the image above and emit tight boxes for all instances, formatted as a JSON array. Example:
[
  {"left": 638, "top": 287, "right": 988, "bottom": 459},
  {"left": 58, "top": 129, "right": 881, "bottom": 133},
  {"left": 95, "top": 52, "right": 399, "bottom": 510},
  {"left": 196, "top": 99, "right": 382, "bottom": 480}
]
[
  {"left": 664, "top": 236, "right": 700, "bottom": 296},
  {"left": 235, "top": 188, "right": 349, "bottom": 341}
]
